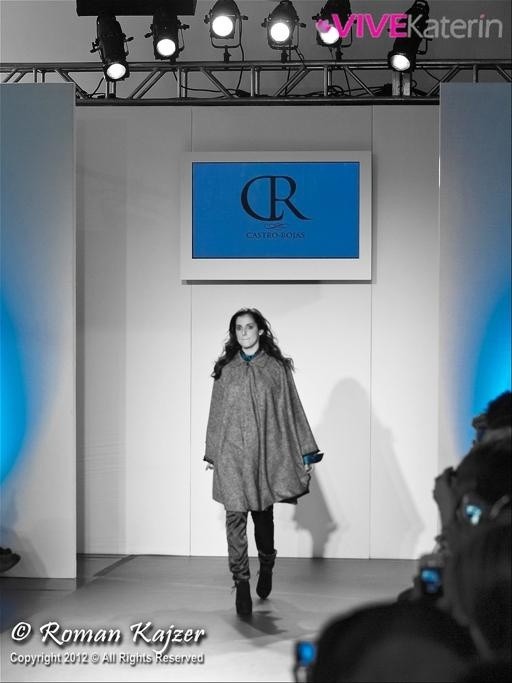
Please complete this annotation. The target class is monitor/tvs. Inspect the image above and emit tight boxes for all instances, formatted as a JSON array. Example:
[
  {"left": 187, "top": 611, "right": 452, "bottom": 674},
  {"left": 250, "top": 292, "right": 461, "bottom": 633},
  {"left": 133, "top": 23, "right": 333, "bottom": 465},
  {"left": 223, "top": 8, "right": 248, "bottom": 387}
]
[
  {"left": 419, "top": 567, "right": 443, "bottom": 598},
  {"left": 465, "top": 503, "right": 482, "bottom": 526}
]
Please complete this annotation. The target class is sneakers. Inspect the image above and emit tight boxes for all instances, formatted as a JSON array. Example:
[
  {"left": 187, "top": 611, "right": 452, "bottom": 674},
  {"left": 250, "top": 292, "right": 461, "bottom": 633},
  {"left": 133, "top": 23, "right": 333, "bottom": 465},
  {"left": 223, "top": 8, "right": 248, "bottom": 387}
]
[
  {"left": 235, "top": 581, "right": 252, "bottom": 615},
  {"left": 256, "top": 571, "right": 272, "bottom": 598}
]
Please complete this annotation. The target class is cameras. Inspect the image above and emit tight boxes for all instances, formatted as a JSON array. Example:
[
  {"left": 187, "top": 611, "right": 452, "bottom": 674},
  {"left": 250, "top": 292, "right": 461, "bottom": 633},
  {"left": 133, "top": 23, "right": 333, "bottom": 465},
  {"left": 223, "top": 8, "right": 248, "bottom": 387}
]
[{"left": 295, "top": 641, "right": 319, "bottom": 666}]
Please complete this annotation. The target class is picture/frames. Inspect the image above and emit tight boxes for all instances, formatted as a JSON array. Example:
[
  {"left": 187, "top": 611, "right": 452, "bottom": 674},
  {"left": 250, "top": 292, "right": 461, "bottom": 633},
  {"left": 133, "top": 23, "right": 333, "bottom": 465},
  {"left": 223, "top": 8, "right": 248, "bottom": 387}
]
[{"left": 181, "top": 147, "right": 373, "bottom": 284}]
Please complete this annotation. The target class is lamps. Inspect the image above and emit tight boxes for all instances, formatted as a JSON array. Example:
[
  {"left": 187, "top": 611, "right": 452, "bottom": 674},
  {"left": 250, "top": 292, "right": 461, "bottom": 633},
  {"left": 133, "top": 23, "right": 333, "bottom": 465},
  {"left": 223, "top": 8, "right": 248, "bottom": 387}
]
[{"left": 91, "top": 0, "right": 431, "bottom": 84}]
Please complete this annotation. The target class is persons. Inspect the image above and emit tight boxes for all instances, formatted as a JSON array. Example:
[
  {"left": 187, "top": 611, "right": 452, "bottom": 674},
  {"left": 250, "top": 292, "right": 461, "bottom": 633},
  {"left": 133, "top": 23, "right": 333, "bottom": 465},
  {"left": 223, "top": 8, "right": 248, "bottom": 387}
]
[
  {"left": 294, "top": 393, "right": 512, "bottom": 682},
  {"left": 200, "top": 307, "right": 323, "bottom": 616}
]
